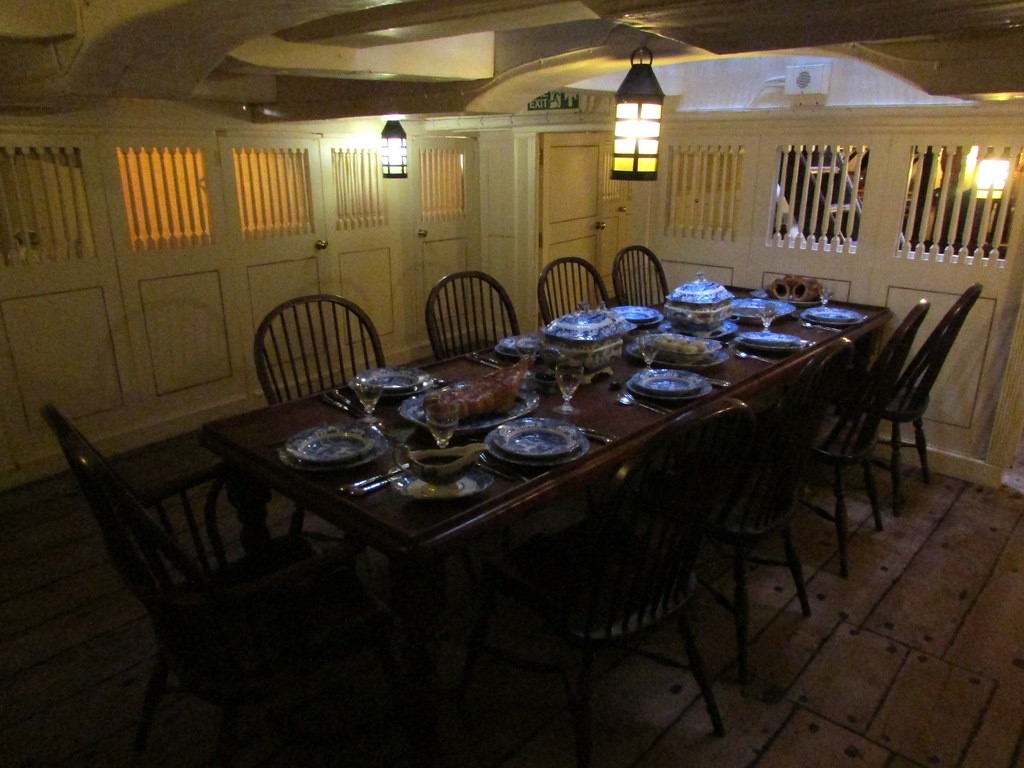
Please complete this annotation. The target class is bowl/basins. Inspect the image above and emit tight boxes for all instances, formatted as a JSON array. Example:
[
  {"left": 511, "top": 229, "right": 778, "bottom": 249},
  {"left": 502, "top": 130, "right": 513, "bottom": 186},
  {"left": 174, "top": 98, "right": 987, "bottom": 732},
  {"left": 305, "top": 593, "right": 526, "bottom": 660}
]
[{"left": 664, "top": 270, "right": 735, "bottom": 334}]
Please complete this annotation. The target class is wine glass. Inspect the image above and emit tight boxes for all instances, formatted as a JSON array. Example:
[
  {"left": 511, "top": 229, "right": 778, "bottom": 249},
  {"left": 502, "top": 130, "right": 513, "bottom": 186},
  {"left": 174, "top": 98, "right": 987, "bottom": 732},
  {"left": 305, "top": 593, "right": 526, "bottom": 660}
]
[
  {"left": 515, "top": 336, "right": 540, "bottom": 373},
  {"left": 818, "top": 288, "right": 834, "bottom": 307},
  {"left": 552, "top": 362, "right": 584, "bottom": 414},
  {"left": 353, "top": 371, "right": 388, "bottom": 431},
  {"left": 637, "top": 329, "right": 662, "bottom": 369},
  {"left": 759, "top": 300, "right": 778, "bottom": 334},
  {"left": 422, "top": 395, "right": 460, "bottom": 449}
]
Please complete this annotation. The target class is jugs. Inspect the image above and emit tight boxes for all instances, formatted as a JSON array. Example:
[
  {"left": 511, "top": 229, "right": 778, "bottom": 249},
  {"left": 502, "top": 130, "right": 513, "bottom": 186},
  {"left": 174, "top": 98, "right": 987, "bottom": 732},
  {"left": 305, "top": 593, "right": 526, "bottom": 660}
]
[{"left": 389, "top": 441, "right": 491, "bottom": 502}]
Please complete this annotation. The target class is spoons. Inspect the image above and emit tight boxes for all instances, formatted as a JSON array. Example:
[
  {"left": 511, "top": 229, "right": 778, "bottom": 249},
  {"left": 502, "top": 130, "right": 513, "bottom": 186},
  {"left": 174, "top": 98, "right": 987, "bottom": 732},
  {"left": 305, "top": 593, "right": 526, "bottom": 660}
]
[{"left": 615, "top": 321, "right": 839, "bottom": 416}]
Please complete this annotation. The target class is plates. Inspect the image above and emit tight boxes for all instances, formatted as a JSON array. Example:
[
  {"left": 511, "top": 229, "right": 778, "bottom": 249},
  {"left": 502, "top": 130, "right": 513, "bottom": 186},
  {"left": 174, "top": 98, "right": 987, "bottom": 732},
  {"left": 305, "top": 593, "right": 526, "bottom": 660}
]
[
  {"left": 608, "top": 290, "right": 864, "bottom": 399},
  {"left": 280, "top": 337, "right": 597, "bottom": 474}
]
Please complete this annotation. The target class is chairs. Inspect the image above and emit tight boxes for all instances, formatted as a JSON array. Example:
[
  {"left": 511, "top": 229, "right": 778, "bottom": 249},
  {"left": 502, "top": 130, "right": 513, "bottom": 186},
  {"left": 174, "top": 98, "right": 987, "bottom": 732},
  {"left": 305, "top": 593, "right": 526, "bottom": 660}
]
[
  {"left": 464, "top": 397, "right": 750, "bottom": 768},
  {"left": 628, "top": 337, "right": 853, "bottom": 685},
  {"left": 254, "top": 294, "right": 388, "bottom": 555},
  {"left": 612, "top": 245, "right": 670, "bottom": 307},
  {"left": 718, "top": 299, "right": 931, "bottom": 578},
  {"left": 425, "top": 270, "right": 520, "bottom": 360},
  {"left": 837, "top": 282, "right": 984, "bottom": 517},
  {"left": 37, "top": 401, "right": 418, "bottom": 768},
  {"left": 538, "top": 257, "right": 610, "bottom": 327}
]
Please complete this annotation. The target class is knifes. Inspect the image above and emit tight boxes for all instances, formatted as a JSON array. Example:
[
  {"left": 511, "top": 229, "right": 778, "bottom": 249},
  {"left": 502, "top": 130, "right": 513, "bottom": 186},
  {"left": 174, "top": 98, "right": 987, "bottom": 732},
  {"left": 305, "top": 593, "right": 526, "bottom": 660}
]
[{"left": 321, "top": 351, "right": 620, "bottom": 494}]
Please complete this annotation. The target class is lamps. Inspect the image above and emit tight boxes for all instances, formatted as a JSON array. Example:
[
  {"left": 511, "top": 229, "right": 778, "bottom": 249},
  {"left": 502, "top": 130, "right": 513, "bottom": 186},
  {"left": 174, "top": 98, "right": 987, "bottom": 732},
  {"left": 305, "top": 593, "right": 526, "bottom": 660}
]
[
  {"left": 609, "top": 39, "right": 665, "bottom": 182},
  {"left": 382, "top": 119, "right": 408, "bottom": 179}
]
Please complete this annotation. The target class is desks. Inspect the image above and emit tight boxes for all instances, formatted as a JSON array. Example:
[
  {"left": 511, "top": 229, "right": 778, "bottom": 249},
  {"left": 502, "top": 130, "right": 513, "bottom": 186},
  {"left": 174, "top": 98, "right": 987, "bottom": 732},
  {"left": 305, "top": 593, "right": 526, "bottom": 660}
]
[{"left": 199, "top": 286, "right": 894, "bottom": 768}]
